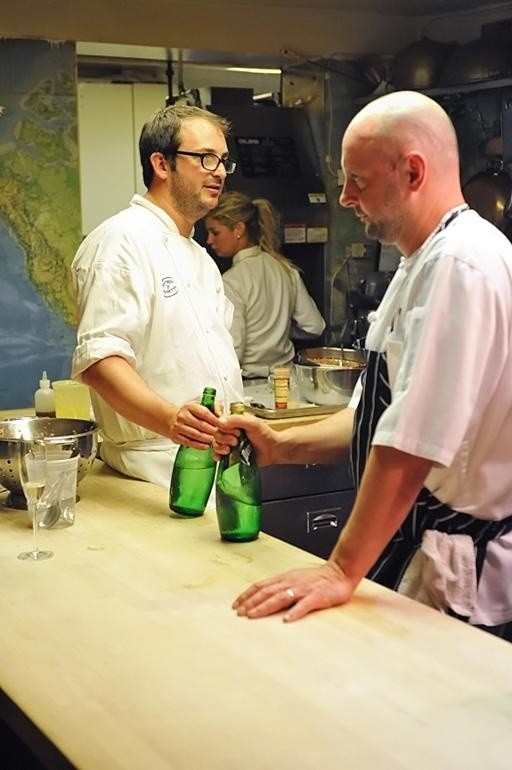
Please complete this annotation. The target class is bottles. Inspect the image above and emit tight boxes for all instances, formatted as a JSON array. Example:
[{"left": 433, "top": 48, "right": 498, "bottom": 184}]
[
  {"left": 167, "top": 385, "right": 218, "bottom": 517},
  {"left": 32, "top": 369, "right": 54, "bottom": 420},
  {"left": 272, "top": 367, "right": 292, "bottom": 410},
  {"left": 215, "top": 402, "right": 262, "bottom": 541}
]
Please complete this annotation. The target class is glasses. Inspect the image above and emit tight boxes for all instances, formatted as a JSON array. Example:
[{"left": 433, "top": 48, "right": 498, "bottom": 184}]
[{"left": 176, "top": 151, "right": 237, "bottom": 175}]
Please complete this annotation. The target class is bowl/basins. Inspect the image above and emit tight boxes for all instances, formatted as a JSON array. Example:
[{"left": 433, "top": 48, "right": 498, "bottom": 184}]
[{"left": 292, "top": 347, "right": 367, "bottom": 407}]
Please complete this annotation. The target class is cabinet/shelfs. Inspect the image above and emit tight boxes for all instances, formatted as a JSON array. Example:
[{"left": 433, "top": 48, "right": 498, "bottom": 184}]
[{"left": 259, "top": 459, "right": 357, "bottom": 561}]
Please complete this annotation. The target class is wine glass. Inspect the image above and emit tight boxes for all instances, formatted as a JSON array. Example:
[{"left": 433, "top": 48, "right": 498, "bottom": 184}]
[{"left": 14, "top": 439, "right": 54, "bottom": 562}]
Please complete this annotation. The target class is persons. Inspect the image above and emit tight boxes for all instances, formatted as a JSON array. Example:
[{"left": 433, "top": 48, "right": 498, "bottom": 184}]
[
  {"left": 199, "top": 88, "right": 510, "bottom": 643},
  {"left": 61, "top": 103, "right": 241, "bottom": 470},
  {"left": 205, "top": 189, "right": 334, "bottom": 379}
]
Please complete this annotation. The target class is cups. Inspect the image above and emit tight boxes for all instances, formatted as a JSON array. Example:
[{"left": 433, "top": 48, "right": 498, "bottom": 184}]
[
  {"left": 51, "top": 376, "right": 93, "bottom": 421},
  {"left": 26, "top": 448, "right": 83, "bottom": 530}
]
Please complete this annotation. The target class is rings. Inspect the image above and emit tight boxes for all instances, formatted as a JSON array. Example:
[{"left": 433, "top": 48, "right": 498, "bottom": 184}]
[{"left": 283, "top": 588, "right": 300, "bottom": 604}]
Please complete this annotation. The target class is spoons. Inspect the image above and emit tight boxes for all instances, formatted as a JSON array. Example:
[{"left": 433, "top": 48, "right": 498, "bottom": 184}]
[{"left": 38, "top": 449, "right": 79, "bottom": 528}]
